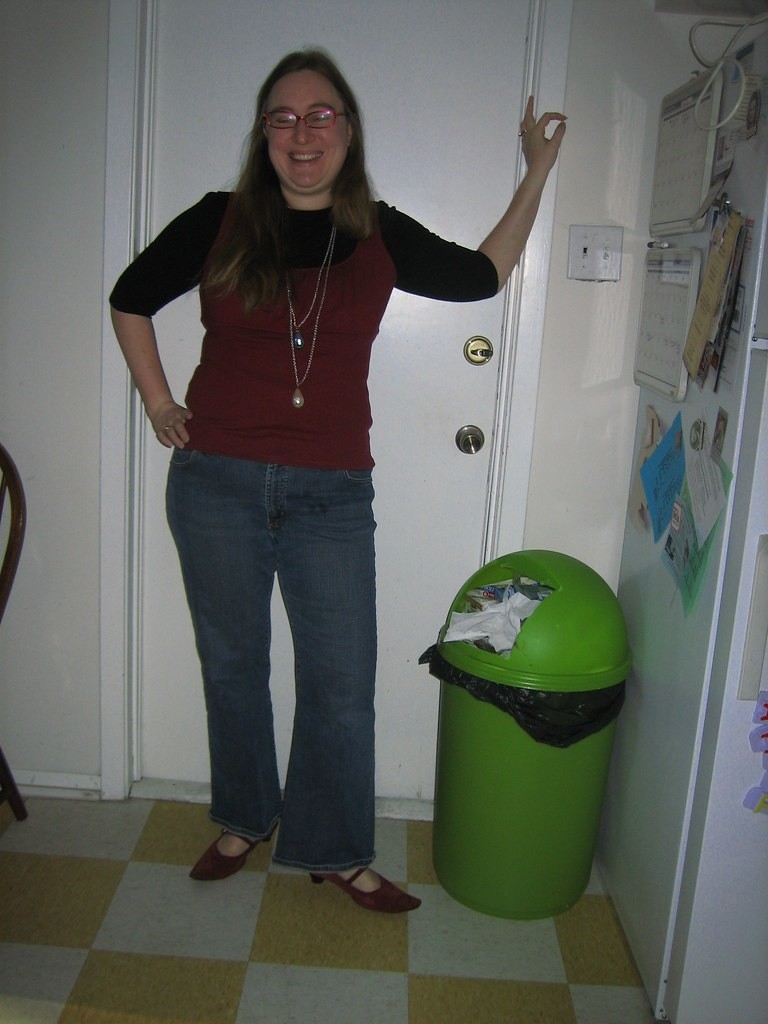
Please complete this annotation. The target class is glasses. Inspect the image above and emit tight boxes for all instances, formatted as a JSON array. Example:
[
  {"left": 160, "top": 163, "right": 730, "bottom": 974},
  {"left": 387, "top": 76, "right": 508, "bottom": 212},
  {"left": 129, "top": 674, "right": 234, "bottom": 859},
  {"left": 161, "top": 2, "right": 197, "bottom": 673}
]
[{"left": 264, "top": 109, "right": 346, "bottom": 129}]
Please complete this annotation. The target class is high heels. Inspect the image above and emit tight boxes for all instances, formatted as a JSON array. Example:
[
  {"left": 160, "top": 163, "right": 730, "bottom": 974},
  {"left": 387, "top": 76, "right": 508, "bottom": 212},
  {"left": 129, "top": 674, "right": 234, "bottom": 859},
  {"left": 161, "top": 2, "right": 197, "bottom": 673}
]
[
  {"left": 189, "top": 819, "right": 279, "bottom": 880},
  {"left": 310, "top": 866, "right": 422, "bottom": 911}
]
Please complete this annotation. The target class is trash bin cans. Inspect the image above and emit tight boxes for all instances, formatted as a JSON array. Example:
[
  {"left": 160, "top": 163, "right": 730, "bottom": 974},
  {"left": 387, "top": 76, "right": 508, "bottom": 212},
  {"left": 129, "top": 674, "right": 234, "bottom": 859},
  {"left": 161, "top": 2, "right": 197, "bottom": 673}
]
[{"left": 418, "top": 549, "right": 633, "bottom": 922}]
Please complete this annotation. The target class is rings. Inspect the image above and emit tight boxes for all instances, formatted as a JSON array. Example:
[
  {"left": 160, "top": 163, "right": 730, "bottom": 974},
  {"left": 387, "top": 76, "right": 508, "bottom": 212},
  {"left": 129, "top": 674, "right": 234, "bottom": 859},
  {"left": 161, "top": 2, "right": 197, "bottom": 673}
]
[
  {"left": 518, "top": 128, "right": 528, "bottom": 137},
  {"left": 164, "top": 425, "right": 169, "bottom": 431}
]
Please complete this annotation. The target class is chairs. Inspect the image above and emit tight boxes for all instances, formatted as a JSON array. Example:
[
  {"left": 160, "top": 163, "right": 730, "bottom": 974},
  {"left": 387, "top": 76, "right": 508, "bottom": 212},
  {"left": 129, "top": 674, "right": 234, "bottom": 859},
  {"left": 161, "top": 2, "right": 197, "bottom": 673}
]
[{"left": 0, "top": 444, "right": 29, "bottom": 826}]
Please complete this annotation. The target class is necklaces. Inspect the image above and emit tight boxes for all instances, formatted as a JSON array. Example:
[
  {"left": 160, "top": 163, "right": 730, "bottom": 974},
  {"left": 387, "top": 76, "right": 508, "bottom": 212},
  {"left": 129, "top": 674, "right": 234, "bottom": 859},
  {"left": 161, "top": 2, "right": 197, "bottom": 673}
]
[{"left": 285, "top": 220, "right": 336, "bottom": 408}]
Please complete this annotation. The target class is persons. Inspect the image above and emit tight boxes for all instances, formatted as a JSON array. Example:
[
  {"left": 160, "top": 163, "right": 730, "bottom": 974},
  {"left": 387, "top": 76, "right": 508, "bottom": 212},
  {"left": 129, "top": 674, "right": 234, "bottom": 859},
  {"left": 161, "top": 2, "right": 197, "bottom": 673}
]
[{"left": 109, "top": 51, "right": 570, "bottom": 914}]
